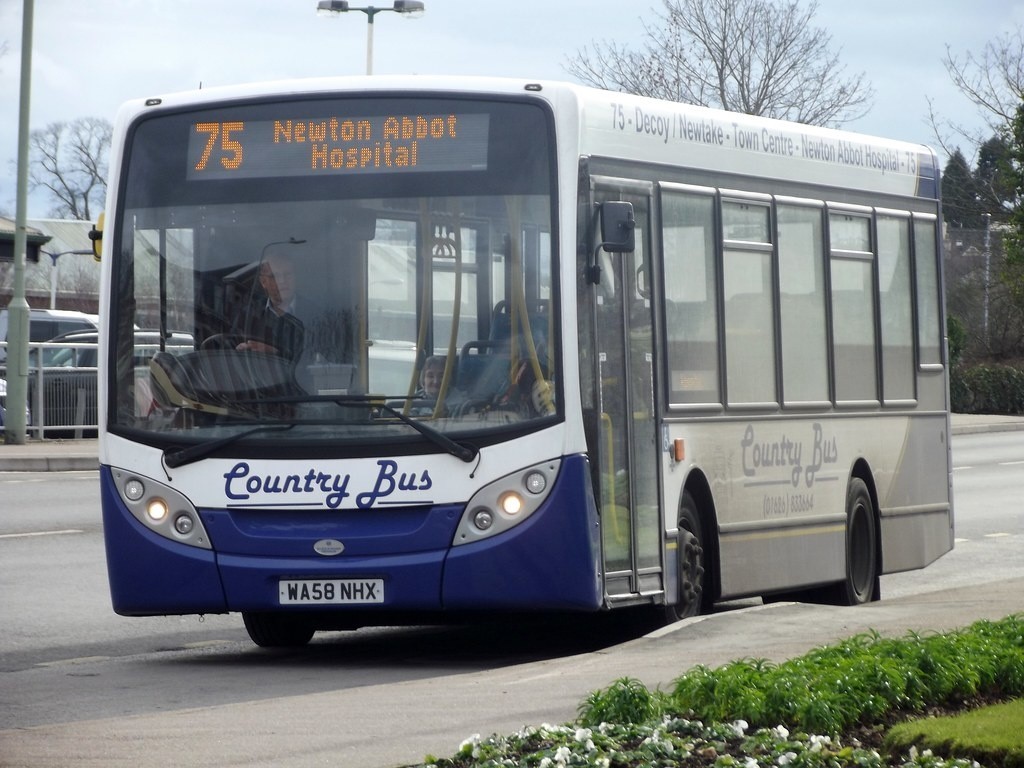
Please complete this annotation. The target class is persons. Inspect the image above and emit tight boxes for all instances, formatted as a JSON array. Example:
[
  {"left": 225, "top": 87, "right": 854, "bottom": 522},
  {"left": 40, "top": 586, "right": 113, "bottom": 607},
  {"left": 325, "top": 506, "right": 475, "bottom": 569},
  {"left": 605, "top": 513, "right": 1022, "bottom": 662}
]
[
  {"left": 409, "top": 356, "right": 474, "bottom": 417},
  {"left": 223, "top": 255, "right": 334, "bottom": 361}
]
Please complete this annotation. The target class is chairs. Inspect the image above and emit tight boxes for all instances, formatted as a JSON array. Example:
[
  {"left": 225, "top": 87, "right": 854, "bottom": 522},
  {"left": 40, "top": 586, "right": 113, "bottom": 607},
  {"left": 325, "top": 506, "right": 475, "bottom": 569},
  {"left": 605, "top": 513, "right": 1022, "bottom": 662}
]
[{"left": 379, "top": 298, "right": 551, "bottom": 418}]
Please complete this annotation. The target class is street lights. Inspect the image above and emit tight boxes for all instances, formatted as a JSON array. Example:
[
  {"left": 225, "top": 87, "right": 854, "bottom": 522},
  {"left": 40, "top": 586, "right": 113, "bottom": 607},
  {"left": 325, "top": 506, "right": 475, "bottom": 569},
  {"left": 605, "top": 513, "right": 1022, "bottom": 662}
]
[{"left": 315, "top": 0, "right": 425, "bottom": 76}]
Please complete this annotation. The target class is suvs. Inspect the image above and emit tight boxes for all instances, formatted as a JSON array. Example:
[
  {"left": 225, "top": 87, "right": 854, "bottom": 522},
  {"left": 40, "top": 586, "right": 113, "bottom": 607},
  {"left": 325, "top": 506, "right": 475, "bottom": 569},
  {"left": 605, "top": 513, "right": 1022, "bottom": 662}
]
[
  {"left": 0, "top": 326, "right": 192, "bottom": 441},
  {"left": 0, "top": 305, "right": 140, "bottom": 358}
]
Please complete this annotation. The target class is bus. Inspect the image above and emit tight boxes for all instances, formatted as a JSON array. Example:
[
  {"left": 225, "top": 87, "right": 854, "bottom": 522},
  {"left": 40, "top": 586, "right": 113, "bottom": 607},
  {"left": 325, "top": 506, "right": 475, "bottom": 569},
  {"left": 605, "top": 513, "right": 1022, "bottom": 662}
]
[{"left": 97, "top": 72, "right": 955, "bottom": 651}]
